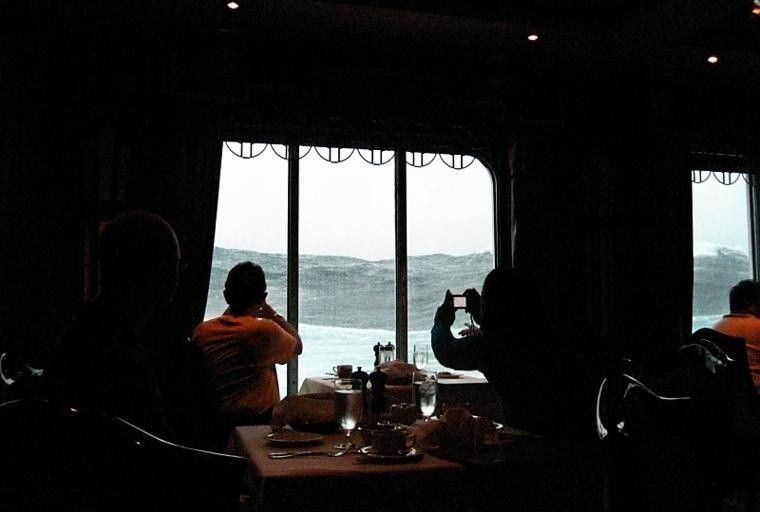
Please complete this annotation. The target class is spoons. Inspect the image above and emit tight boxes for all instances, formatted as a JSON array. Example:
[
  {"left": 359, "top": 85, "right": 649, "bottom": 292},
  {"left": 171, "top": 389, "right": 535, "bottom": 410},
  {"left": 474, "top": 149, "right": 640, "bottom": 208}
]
[{"left": 268, "top": 441, "right": 353, "bottom": 460}]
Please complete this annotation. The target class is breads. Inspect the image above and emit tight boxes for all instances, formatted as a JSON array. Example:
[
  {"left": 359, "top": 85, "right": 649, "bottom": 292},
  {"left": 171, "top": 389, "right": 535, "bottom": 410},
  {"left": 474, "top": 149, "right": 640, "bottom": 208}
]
[{"left": 417, "top": 408, "right": 498, "bottom": 454}]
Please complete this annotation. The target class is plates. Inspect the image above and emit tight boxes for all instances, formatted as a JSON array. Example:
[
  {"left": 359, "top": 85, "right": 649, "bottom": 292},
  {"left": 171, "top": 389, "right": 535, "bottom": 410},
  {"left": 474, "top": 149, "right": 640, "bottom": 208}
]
[
  {"left": 265, "top": 431, "right": 324, "bottom": 443},
  {"left": 436, "top": 372, "right": 464, "bottom": 379},
  {"left": 358, "top": 445, "right": 423, "bottom": 461}
]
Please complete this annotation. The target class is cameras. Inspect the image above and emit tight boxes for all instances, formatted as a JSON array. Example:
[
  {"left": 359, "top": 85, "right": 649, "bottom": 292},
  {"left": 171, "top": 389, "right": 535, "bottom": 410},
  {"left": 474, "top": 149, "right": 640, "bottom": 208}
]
[{"left": 452, "top": 295, "right": 467, "bottom": 309}]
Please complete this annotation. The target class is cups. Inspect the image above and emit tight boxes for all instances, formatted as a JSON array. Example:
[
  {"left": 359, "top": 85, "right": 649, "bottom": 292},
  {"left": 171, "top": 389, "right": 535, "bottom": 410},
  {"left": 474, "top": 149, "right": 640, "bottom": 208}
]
[
  {"left": 333, "top": 365, "right": 353, "bottom": 378},
  {"left": 372, "top": 430, "right": 418, "bottom": 455},
  {"left": 412, "top": 369, "right": 439, "bottom": 420},
  {"left": 413, "top": 343, "right": 428, "bottom": 371}
]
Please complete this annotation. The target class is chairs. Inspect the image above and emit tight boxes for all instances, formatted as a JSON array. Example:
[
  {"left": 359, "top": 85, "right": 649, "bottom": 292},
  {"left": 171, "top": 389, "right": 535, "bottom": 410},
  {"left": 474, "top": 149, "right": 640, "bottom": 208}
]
[{"left": 692, "top": 328, "right": 759, "bottom": 400}]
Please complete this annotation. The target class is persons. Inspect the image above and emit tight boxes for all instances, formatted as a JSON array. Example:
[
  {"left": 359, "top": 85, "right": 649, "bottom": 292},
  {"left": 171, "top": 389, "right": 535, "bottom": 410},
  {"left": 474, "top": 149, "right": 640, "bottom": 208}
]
[
  {"left": 432, "top": 266, "right": 575, "bottom": 437},
  {"left": 191, "top": 262, "right": 302, "bottom": 425},
  {"left": 713, "top": 279, "right": 760, "bottom": 393},
  {"left": 45, "top": 209, "right": 234, "bottom": 455}
]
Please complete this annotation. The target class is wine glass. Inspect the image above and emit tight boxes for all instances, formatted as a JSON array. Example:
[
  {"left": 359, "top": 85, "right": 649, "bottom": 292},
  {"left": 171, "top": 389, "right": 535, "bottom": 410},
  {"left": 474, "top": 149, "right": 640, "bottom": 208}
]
[{"left": 332, "top": 379, "right": 365, "bottom": 450}]
